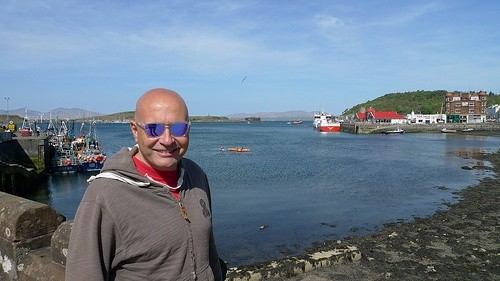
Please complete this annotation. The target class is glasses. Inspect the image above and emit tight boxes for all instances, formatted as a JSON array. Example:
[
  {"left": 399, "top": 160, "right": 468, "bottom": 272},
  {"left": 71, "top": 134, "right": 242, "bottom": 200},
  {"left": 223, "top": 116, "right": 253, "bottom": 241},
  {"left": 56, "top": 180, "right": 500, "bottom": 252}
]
[{"left": 136, "top": 122, "right": 190, "bottom": 138}]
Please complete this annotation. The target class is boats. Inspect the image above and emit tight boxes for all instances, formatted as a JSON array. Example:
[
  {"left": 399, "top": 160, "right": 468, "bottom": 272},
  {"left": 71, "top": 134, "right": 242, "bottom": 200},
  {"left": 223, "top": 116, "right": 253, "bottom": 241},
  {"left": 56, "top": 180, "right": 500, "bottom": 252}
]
[
  {"left": 381, "top": 129, "right": 404, "bottom": 135},
  {"left": 441, "top": 128, "right": 474, "bottom": 133},
  {"left": 312, "top": 112, "right": 340, "bottom": 132},
  {"left": 19, "top": 108, "right": 107, "bottom": 175},
  {"left": 228, "top": 146, "right": 251, "bottom": 152},
  {"left": 286, "top": 119, "right": 303, "bottom": 125}
]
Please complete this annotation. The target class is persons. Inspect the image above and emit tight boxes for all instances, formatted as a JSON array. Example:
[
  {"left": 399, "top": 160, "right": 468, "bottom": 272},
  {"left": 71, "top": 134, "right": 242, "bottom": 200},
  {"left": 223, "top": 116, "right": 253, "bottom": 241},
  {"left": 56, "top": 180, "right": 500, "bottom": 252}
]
[{"left": 65, "top": 88, "right": 227, "bottom": 281}]
